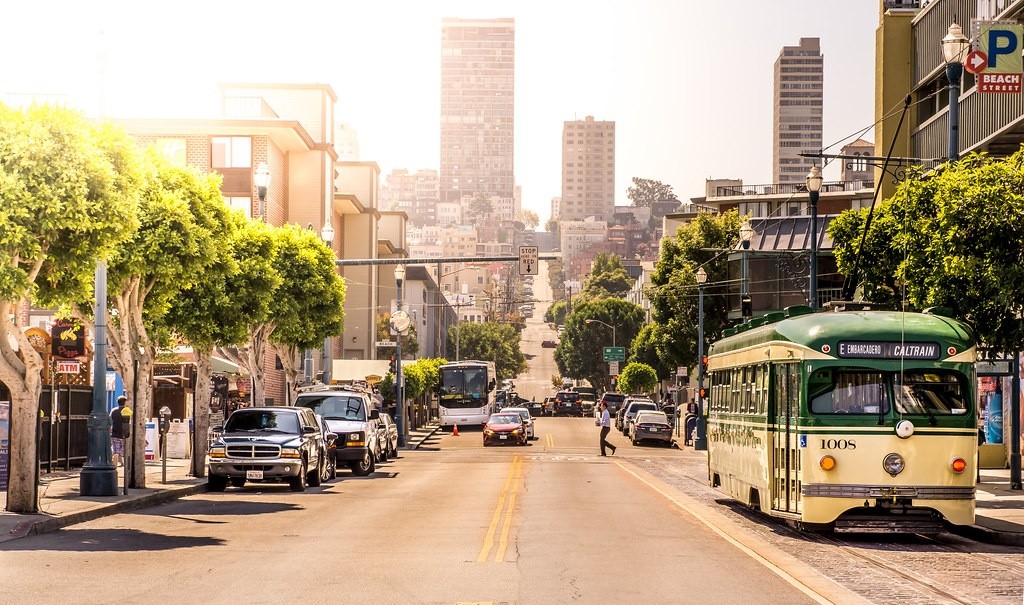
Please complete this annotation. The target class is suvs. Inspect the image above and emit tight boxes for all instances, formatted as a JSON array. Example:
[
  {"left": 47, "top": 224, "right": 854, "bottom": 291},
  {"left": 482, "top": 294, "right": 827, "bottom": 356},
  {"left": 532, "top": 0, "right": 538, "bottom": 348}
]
[
  {"left": 542, "top": 340, "right": 559, "bottom": 348},
  {"left": 293, "top": 391, "right": 387, "bottom": 476},
  {"left": 543, "top": 386, "right": 625, "bottom": 418}
]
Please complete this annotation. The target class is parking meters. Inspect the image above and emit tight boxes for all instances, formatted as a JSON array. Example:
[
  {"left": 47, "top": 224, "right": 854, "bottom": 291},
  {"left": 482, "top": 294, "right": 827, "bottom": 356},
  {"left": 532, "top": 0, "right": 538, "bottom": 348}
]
[
  {"left": 158, "top": 406, "right": 172, "bottom": 483},
  {"left": 121, "top": 407, "right": 133, "bottom": 495}
]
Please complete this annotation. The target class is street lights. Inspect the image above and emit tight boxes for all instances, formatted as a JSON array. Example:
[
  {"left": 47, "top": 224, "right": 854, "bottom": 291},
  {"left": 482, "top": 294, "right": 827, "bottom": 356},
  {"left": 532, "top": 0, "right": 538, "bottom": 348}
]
[
  {"left": 254, "top": 161, "right": 271, "bottom": 222},
  {"left": 739, "top": 224, "right": 754, "bottom": 317},
  {"left": 394, "top": 263, "right": 404, "bottom": 443},
  {"left": 320, "top": 219, "right": 335, "bottom": 383},
  {"left": 940, "top": 14, "right": 969, "bottom": 163},
  {"left": 585, "top": 319, "right": 616, "bottom": 387},
  {"left": 804, "top": 161, "right": 824, "bottom": 312},
  {"left": 435, "top": 266, "right": 480, "bottom": 360},
  {"left": 694, "top": 264, "right": 709, "bottom": 450},
  {"left": 456, "top": 297, "right": 492, "bottom": 361}
]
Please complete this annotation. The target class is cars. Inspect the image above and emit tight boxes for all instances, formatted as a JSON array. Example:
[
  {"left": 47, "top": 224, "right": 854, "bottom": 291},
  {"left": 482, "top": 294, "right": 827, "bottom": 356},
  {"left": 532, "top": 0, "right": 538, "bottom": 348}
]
[
  {"left": 519, "top": 275, "right": 535, "bottom": 327},
  {"left": 496, "top": 380, "right": 518, "bottom": 412},
  {"left": 615, "top": 394, "right": 674, "bottom": 445},
  {"left": 207, "top": 406, "right": 338, "bottom": 491},
  {"left": 517, "top": 402, "right": 545, "bottom": 416},
  {"left": 378, "top": 412, "right": 399, "bottom": 461},
  {"left": 481, "top": 408, "right": 537, "bottom": 446},
  {"left": 556, "top": 325, "right": 564, "bottom": 338}
]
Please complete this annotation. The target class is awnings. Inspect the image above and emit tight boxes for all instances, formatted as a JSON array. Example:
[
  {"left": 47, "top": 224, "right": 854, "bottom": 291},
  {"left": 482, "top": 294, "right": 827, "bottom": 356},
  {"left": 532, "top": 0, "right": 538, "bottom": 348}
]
[{"left": 211, "top": 356, "right": 249, "bottom": 374}]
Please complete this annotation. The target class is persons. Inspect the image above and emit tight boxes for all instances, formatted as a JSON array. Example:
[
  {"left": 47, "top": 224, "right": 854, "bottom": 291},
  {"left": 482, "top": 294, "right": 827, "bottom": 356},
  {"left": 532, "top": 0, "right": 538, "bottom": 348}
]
[
  {"left": 597, "top": 402, "right": 616, "bottom": 456},
  {"left": 687, "top": 398, "right": 698, "bottom": 415},
  {"left": 374, "top": 388, "right": 385, "bottom": 412},
  {"left": 109, "top": 395, "right": 130, "bottom": 468}
]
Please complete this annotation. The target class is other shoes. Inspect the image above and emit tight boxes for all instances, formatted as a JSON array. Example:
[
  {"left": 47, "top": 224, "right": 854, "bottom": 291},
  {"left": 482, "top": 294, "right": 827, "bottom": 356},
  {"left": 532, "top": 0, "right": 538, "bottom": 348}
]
[
  {"left": 611, "top": 447, "right": 617, "bottom": 455},
  {"left": 598, "top": 453, "right": 606, "bottom": 457}
]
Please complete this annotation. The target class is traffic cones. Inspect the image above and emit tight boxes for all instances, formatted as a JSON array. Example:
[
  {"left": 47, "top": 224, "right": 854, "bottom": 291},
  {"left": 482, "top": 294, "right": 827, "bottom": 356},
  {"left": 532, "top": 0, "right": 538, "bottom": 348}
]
[{"left": 452, "top": 421, "right": 460, "bottom": 436}]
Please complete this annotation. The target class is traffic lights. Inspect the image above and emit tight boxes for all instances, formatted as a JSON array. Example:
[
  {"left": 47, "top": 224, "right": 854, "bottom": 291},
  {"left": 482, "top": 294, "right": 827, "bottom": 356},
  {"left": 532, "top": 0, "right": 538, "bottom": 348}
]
[
  {"left": 390, "top": 355, "right": 396, "bottom": 374},
  {"left": 740, "top": 292, "right": 752, "bottom": 316},
  {"left": 701, "top": 354, "right": 708, "bottom": 364},
  {"left": 700, "top": 388, "right": 708, "bottom": 397}
]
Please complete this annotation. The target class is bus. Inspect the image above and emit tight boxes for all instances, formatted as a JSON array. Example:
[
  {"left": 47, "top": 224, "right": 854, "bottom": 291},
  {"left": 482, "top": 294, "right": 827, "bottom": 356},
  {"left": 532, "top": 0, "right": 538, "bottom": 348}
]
[
  {"left": 705, "top": 93, "right": 977, "bottom": 536},
  {"left": 438, "top": 361, "right": 497, "bottom": 430}
]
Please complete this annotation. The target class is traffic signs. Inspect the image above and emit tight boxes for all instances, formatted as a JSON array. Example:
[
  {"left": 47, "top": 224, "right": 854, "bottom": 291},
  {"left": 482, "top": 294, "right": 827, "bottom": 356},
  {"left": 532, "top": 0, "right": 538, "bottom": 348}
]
[
  {"left": 604, "top": 347, "right": 625, "bottom": 363},
  {"left": 966, "top": 24, "right": 1024, "bottom": 93},
  {"left": 519, "top": 246, "right": 538, "bottom": 275}
]
